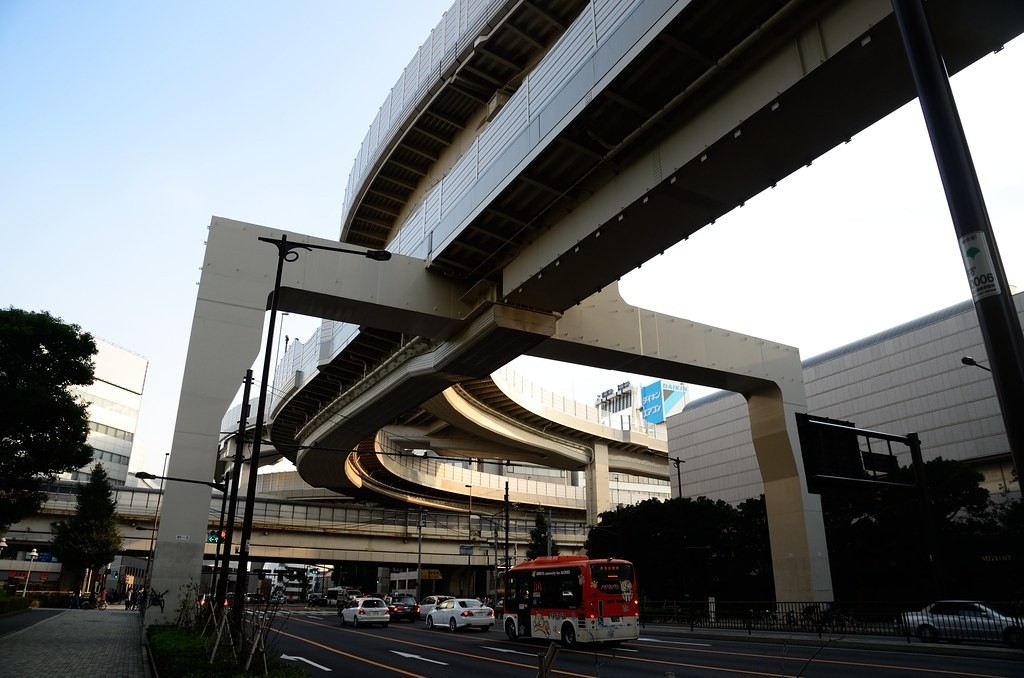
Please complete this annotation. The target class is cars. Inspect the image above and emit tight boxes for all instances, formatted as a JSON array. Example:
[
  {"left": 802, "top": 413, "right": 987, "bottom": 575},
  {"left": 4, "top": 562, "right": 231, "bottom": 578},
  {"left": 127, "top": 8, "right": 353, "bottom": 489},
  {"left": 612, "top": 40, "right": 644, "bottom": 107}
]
[
  {"left": 418, "top": 595, "right": 456, "bottom": 618},
  {"left": 901, "top": 600, "right": 1024, "bottom": 647},
  {"left": 341, "top": 597, "right": 391, "bottom": 628},
  {"left": 426, "top": 598, "right": 495, "bottom": 633},
  {"left": 243, "top": 592, "right": 285, "bottom": 605}
]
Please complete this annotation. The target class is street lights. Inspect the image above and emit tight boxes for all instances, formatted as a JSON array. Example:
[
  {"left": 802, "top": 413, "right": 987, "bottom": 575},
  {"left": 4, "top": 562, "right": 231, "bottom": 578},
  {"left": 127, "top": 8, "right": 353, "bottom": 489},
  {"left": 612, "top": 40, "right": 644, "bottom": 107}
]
[
  {"left": 132, "top": 471, "right": 228, "bottom": 606},
  {"left": 22, "top": 548, "right": 38, "bottom": 598},
  {"left": 465, "top": 484, "right": 472, "bottom": 598},
  {"left": 640, "top": 449, "right": 686, "bottom": 497},
  {"left": 228, "top": 232, "right": 395, "bottom": 642}
]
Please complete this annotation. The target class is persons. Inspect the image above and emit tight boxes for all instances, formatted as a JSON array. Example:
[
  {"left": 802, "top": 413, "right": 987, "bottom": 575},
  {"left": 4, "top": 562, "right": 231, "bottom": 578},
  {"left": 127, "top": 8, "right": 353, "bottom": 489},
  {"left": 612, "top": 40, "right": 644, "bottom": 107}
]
[
  {"left": 73, "top": 585, "right": 82, "bottom": 609},
  {"left": 100, "top": 586, "right": 144, "bottom": 611},
  {"left": 475, "top": 596, "right": 504, "bottom": 619}
]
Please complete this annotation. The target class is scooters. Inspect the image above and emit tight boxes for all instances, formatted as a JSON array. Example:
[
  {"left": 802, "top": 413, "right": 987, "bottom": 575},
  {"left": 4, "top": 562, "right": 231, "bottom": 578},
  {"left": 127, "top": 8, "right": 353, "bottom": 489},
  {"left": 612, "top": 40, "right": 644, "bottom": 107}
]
[{"left": 80, "top": 599, "right": 108, "bottom": 611}]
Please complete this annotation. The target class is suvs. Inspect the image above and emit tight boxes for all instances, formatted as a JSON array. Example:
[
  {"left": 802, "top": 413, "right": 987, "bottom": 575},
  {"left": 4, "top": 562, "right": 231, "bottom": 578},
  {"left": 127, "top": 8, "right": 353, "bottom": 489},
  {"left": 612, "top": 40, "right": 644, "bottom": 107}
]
[
  {"left": 308, "top": 593, "right": 327, "bottom": 608},
  {"left": 383, "top": 595, "right": 420, "bottom": 623}
]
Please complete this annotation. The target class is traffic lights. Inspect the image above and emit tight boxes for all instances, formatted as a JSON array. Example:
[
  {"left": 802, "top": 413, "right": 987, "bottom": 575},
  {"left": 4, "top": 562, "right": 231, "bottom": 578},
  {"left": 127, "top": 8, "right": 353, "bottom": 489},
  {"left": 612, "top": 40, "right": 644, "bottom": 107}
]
[
  {"left": 209, "top": 530, "right": 226, "bottom": 543},
  {"left": 311, "top": 568, "right": 320, "bottom": 575},
  {"left": 114, "top": 573, "right": 118, "bottom": 579}
]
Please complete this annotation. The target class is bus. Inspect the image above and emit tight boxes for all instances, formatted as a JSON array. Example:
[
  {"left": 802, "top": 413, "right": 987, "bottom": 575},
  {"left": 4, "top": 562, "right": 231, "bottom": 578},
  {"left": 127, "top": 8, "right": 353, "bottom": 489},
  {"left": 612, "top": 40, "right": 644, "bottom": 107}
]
[
  {"left": 502, "top": 555, "right": 641, "bottom": 649},
  {"left": 326, "top": 587, "right": 363, "bottom": 608}
]
